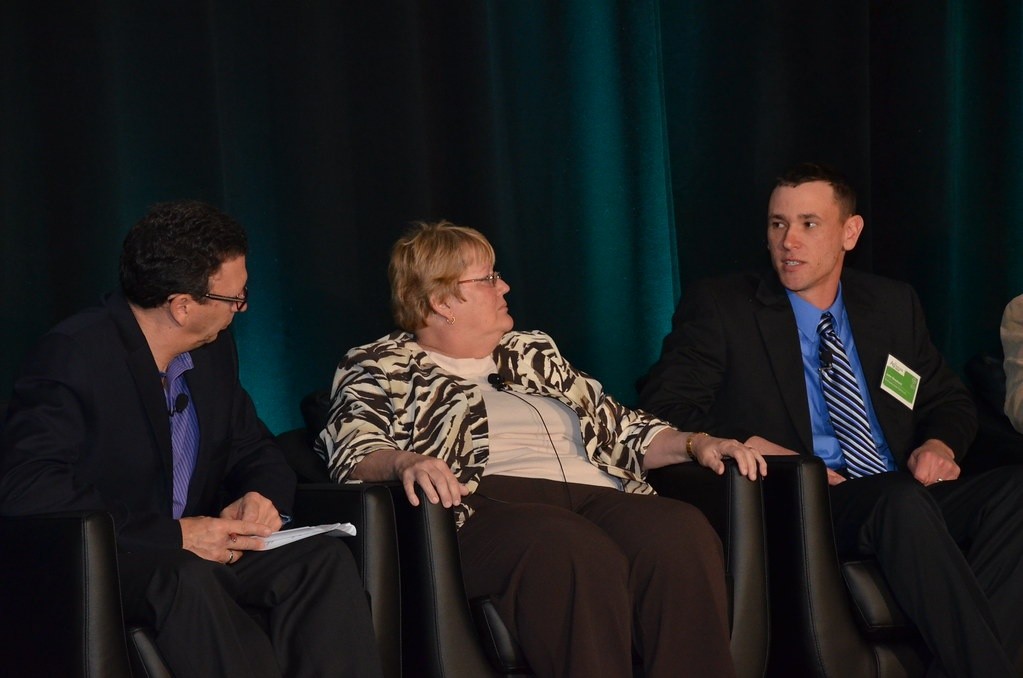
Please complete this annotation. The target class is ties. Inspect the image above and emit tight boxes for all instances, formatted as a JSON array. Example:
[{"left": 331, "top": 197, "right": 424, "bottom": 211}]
[{"left": 815, "top": 314, "right": 887, "bottom": 480}]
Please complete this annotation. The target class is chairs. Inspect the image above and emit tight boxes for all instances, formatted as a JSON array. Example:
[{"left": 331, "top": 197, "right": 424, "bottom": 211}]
[
  {"left": 279, "top": 388, "right": 769, "bottom": 677},
  {"left": 0, "top": 477, "right": 400, "bottom": 678},
  {"left": 748, "top": 451, "right": 938, "bottom": 677}
]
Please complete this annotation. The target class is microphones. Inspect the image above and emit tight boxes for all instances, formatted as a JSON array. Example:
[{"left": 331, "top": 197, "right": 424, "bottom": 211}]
[
  {"left": 167, "top": 392, "right": 188, "bottom": 418},
  {"left": 488, "top": 372, "right": 506, "bottom": 393},
  {"left": 817, "top": 351, "right": 834, "bottom": 372}
]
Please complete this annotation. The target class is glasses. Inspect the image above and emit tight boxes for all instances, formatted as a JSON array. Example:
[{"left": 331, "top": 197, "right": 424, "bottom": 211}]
[
  {"left": 458, "top": 271, "right": 502, "bottom": 286},
  {"left": 204, "top": 285, "right": 249, "bottom": 310}
]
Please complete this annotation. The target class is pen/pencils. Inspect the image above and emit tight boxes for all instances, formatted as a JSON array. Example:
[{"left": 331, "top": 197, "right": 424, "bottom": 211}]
[{"left": 228, "top": 532, "right": 239, "bottom": 546}]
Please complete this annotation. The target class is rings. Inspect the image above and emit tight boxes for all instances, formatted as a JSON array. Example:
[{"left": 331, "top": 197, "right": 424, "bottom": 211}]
[
  {"left": 225, "top": 549, "right": 233, "bottom": 564},
  {"left": 747, "top": 446, "right": 754, "bottom": 450},
  {"left": 937, "top": 477, "right": 944, "bottom": 482}
]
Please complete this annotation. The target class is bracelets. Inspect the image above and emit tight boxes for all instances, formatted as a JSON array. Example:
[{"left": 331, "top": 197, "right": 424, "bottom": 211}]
[{"left": 686, "top": 431, "right": 710, "bottom": 462}]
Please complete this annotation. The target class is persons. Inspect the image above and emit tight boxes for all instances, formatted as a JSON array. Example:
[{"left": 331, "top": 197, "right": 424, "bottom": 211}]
[
  {"left": 641, "top": 164, "right": 1023, "bottom": 678},
  {"left": 999, "top": 294, "right": 1023, "bottom": 435},
  {"left": 0, "top": 199, "right": 386, "bottom": 678},
  {"left": 312, "top": 219, "right": 768, "bottom": 678}
]
